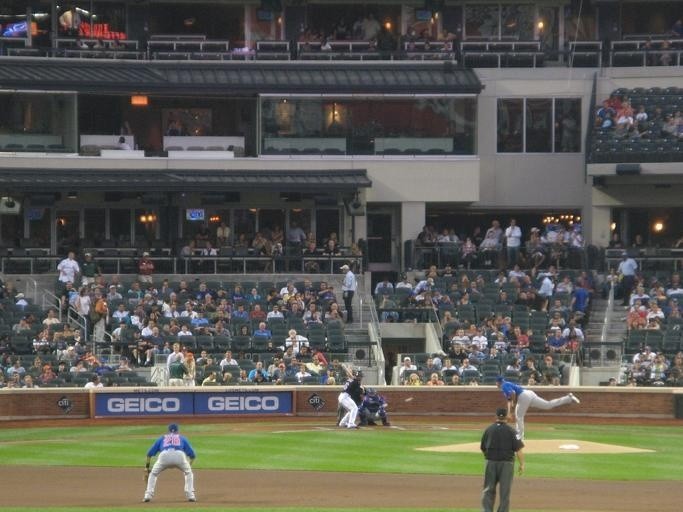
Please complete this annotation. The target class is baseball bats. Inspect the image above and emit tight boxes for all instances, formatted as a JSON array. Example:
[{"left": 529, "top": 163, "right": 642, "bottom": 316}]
[{"left": 342, "top": 364, "right": 353, "bottom": 379}]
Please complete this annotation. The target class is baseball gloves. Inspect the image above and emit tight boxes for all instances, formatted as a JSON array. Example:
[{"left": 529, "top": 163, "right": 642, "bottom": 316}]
[
  {"left": 508, "top": 414, "right": 516, "bottom": 422},
  {"left": 144, "top": 469, "right": 151, "bottom": 483}
]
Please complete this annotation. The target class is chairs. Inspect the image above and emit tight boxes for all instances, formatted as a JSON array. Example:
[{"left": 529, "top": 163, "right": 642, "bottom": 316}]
[{"left": 590, "top": 87, "right": 682, "bottom": 162}]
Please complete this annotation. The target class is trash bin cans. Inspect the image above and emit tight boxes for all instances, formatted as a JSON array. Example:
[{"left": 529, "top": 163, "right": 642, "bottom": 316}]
[{"left": 675, "top": 394, "right": 683, "bottom": 418}]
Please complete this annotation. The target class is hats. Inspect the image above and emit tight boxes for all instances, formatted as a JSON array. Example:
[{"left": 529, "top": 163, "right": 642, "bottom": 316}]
[
  {"left": 169, "top": 424, "right": 178, "bottom": 430},
  {"left": 496, "top": 408, "right": 507, "bottom": 416},
  {"left": 340, "top": 265, "right": 350, "bottom": 269}
]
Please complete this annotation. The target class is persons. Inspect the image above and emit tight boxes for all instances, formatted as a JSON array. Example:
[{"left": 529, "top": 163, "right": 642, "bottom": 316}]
[
  {"left": 606, "top": 346, "right": 681, "bottom": 388},
  {"left": 602, "top": 266, "right": 680, "bottom": 333},
  {"left": 79, "top": 252, "right": 102, "bottom": 287},
  {"left": 2, "top": 274, "right": 354, "bottom": 387},
  {"left": 59, "top": 2, "right": 81, "bottom": 56},
  {"left": 338, "top": 264, "right": 358, "bottom": 324},
  {"left": 336, "top": 368, "right": 390, "bottom": 429},
  {"left": 616, "top": 252, "right": 638, "bottom": 306},
  {"left": 166, "top": 120, "right": 179, "bottom": 136},
  {"left": 593, "top": 95, "right": 683, "bottom": 144},
  {"left": 306, "top": 12, "right": 381, "bottom": 60},
  {"left": 374, "top": 272, "right": 435, "bottom": 323},
  {"left": 481, "top": 408, "right": 524, "bottom": 512},
  {"left": 496, "top": 376, "right": 580, "bottom": 440},
  {"left": 137, "top": 252, "right": 154, "bottom": 284},
  {"left": 424, "top": 265, "right": 606, "bottom": 365},
  {"left": 504, "top": 355, "right": 563, "bottom": 388},
  {"left": 561, "top": 112, "right": 577, "bottom": 152},
  {"left": 643, "top": 19, "right": 683, "bottom": 66},
  {"left": 57, "top": 252, "right": 80, "bottom": 310},
  {"left": 143, "top": 423, "right": 197, "bottom": 502},
  {"left": 2, "top": 220, "right": 362, "bottom": 274},
  {"left": 114, "top": 118, "right": 132, "bottom": 150},
  {"left": 412, "top": 219, "right": 681, "bottom": 269},
  {"left": 406, "top": 27, "right": 457, "bottom": 60},
  {"left": 400, "top": 353, "right": 483, "bottom": 387}
]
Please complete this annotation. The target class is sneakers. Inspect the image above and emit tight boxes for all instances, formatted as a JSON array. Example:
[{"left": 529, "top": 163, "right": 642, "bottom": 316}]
[
  {"left": 142, "top": 497, "right": 150, "bottom": 502},
  {"left": 187, "top": 496, "right": 196, "bottom": 501},
  {"left": 339, "top": 421, "right": 391, "bottom": 428},
  {"left": 569, "top": 392, "right": 579, "bottom": 404}
]
